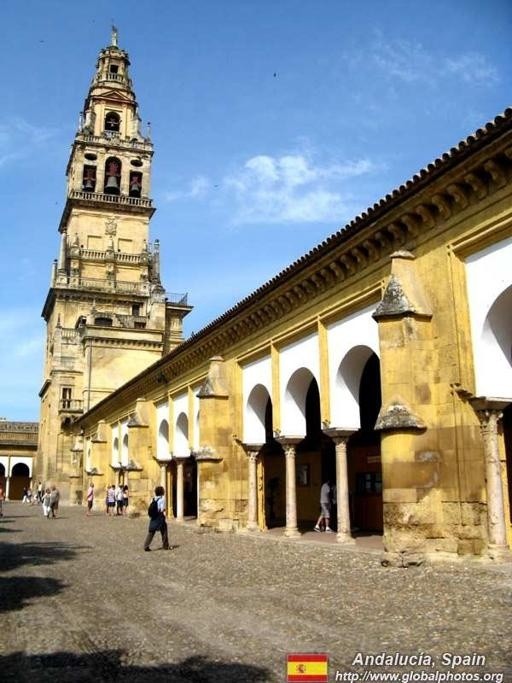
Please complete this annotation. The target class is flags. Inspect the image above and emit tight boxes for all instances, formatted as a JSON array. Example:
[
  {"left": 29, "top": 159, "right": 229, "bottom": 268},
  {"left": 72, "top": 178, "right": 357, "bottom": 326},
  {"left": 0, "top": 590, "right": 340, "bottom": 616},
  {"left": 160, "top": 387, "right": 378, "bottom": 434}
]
[{"left": 285, "top": 653, "right": 329, "bottom": 682}]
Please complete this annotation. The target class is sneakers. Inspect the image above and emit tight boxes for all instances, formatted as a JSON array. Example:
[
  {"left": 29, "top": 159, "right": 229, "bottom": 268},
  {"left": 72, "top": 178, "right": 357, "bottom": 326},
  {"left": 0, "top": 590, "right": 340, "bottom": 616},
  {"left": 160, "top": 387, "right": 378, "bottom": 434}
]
[
  {"left": 314, "top": 527, "right": 335, "bottom": 534},
  {"left": 145, "top": 547, "right": 152, "bottom": 550}
]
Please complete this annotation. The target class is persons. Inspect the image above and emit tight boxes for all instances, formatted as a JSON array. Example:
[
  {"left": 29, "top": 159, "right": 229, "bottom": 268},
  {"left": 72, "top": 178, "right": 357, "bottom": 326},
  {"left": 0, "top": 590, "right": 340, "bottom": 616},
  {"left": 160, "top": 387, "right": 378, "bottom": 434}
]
[
  {"left": 50, "top": 483, "right": 60, "bottom": 518},
  {"left": 106, "top": 484, "right": 130, "bottom": 516},
  {"left": 0, "top": 480, "right": 6, "bottom": 516},
  {"left": 21, "top": 481, "right": 44, "bottom": 504},
  {"left": 86, "top": 482, "right": 95, "bottom": 515},
  {"left": 143, "top": 485, "right": 175, "bottom": 550},
  {"left": 41, "top": 488, "right": 51, "bottom": 518},
  {"left": 312, "top": 476, "right": 337, "bottom": 534}
]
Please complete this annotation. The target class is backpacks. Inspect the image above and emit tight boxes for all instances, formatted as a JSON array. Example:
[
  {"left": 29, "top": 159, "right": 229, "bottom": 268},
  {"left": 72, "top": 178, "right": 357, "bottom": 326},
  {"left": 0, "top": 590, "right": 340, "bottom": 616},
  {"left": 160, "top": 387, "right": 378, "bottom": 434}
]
[{"left": 148, "top": 497, "right": 160, "bottom": 519}]
